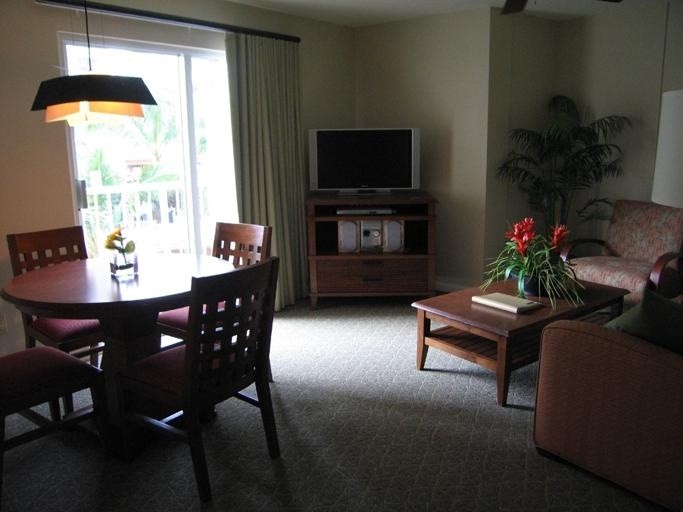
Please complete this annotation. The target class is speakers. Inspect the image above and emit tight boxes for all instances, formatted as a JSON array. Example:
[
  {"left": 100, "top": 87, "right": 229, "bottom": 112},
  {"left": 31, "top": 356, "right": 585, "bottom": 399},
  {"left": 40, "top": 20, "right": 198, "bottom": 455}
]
[{"left": 338, "top": 220, "right": 405, "bottom": 254}]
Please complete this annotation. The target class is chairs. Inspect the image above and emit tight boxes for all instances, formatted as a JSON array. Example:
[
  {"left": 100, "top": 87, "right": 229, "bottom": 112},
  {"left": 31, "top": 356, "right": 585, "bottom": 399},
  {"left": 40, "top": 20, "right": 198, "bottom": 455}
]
[
  {"left": 7, "top": 227, "right": 106, "bottom": 416},
  {"left": 121, "top": 255, "right": 280, "bottom": 512},
  {"left": 155, "top": 223, "right": 273, "bottom": 419},
  {"left": 0, "top": 346, "right": 130, "bottom": 484},
  {"left": 558, "top": 199, "right": 683, "bottom": 311}
]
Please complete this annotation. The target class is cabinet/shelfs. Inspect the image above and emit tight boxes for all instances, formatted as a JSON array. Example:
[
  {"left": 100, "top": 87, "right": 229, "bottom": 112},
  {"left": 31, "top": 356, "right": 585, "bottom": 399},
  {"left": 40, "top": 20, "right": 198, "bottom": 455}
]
[{"left": 304, "top": 195, "right": 437, "bottom": 311}]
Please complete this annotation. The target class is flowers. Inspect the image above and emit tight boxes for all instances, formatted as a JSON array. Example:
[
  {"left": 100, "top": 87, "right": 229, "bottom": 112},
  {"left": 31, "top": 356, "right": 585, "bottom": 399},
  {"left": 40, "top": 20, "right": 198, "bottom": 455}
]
[
  {"left": 481, "top": 218, "right": 586, "bottom": 311},
  {"left": 103, "top": 227, "right": 136, "bottom": 264}
]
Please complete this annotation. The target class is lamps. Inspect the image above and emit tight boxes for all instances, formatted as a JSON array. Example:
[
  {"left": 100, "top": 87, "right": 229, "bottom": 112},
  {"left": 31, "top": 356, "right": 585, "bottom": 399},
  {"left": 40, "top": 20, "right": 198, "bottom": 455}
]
[{"left": 31, "top": 0, "right": 156, "bottom": 128}]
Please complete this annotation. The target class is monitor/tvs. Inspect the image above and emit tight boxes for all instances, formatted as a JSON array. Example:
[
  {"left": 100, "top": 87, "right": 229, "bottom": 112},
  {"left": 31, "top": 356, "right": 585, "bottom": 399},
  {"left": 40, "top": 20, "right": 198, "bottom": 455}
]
[{"left": 308, "top": 128, "right": 421, "bottom": 195}]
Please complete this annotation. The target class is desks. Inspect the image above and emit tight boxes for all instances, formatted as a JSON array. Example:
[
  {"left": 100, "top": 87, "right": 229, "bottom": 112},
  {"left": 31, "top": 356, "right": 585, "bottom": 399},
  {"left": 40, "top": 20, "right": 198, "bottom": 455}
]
[{"left": 0, "top": 256, "right": 235, "bottom": 431}]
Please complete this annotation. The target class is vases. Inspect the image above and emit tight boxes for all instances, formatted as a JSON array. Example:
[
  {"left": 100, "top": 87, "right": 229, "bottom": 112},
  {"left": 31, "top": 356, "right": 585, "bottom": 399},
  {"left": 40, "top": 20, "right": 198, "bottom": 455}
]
[
  {"left": 111, "top": 256, "right": 138, "bottom": 277},
  {"left": 518, "top": 273, "right": 551, "bottom": 296}
]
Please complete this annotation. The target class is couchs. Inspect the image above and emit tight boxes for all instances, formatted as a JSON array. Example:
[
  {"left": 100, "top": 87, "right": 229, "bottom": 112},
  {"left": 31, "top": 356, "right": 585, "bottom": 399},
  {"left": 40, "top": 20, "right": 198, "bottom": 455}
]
[{"left": 535, "top": 288, "right": 683, "bottom": 512}]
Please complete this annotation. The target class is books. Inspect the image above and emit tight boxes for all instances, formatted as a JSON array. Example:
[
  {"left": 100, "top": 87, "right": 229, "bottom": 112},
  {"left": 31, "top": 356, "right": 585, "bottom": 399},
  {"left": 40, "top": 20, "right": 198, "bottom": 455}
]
[{"left": 471, "top": 292, "right": 545, "bottom": 315}]
